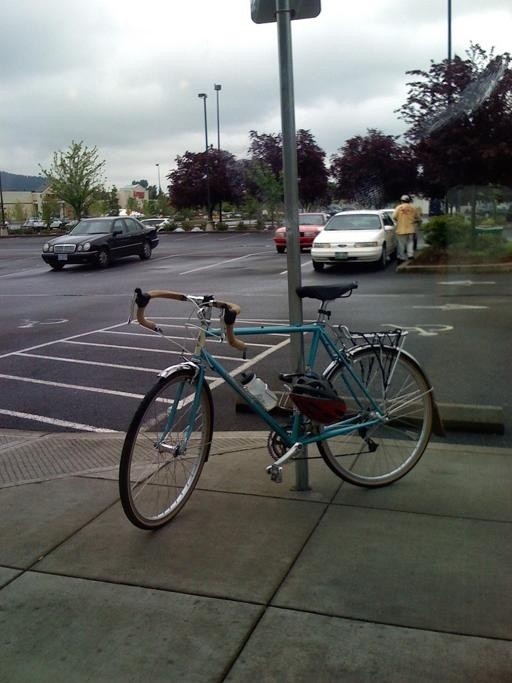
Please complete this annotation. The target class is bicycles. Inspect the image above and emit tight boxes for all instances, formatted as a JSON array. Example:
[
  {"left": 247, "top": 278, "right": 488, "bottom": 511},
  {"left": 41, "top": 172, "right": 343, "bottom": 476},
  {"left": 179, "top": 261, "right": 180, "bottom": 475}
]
[{"left": 115, "top": 282, "right": 447, "bottom": 530}]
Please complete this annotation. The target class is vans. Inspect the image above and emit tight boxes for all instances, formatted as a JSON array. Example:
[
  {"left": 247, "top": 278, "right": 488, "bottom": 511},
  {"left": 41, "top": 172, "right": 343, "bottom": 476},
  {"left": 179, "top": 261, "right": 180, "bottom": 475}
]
[{"left": 139, "top": 218, "right": 170, "bottom": 231}]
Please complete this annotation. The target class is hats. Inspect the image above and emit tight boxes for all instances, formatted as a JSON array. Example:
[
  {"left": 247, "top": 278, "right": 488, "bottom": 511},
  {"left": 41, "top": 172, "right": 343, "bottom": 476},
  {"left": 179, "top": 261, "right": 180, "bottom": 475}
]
[{"left": 400, "top": 195, "right": 409, "bottom": 201}]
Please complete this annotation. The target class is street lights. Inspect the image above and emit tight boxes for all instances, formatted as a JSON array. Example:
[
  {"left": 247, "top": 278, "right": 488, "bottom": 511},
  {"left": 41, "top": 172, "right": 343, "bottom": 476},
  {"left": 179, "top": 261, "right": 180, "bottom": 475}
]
[
  {"left": 198, "top": 94, "right": 213, "bottom": 222},
  {"left": 155, "top": 163, "right": 162, "bottom": 193},
  {"left": 214, "top": 83, "right": 223, "bottom": 222}
]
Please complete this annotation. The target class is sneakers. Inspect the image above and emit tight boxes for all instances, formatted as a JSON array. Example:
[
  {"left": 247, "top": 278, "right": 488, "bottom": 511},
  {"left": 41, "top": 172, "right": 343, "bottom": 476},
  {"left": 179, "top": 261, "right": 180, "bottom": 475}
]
[{"left": 395, "top": 256, "right": 416, "bottom": 265}]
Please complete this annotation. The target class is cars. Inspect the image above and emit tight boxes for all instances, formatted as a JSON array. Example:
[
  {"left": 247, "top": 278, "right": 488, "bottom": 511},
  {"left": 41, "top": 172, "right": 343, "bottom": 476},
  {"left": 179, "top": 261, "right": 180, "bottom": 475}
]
[
  {"left": 25, "top": 215, "right": 63, "bottom": 230},
  {"left": 41, "top": 215, "right": 160, "bottom": 270},
  {"left": 273, "top": 212, "right": 327, "bottom": 253},
  {"left": 310, "top": 209, "right": 398, "bottom": 271}
]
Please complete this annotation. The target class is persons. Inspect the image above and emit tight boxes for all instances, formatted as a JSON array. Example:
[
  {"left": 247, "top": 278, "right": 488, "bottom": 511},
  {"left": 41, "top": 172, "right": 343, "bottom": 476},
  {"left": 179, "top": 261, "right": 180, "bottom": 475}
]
[{"left": 392, "top": 194, "right": 419, "bottom": 266}]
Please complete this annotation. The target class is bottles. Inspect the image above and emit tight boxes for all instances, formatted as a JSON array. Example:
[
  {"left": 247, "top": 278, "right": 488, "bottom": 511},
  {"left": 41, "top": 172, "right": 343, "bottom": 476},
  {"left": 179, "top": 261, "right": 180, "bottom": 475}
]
[{"left": 239, "top": 371, "right": 278, "bottom": 412}]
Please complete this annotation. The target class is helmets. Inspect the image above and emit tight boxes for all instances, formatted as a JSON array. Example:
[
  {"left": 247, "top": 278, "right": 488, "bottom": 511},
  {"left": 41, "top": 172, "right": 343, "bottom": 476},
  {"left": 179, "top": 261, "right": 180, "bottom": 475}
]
[{"left": 289, "top": 376, "right": 347, "bottom": 424}]
